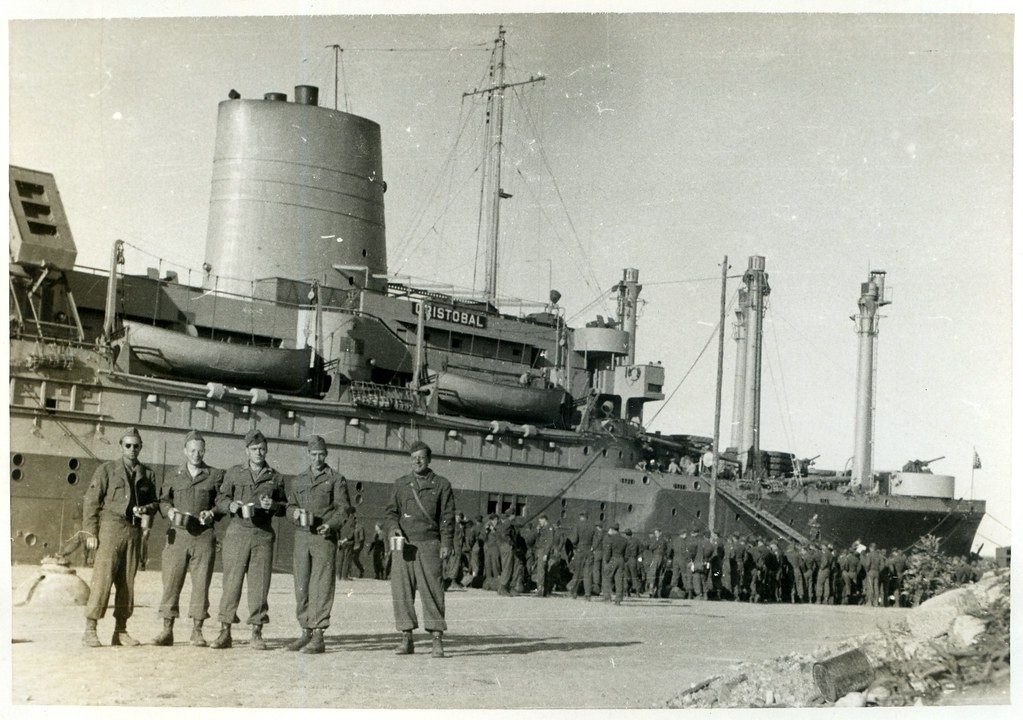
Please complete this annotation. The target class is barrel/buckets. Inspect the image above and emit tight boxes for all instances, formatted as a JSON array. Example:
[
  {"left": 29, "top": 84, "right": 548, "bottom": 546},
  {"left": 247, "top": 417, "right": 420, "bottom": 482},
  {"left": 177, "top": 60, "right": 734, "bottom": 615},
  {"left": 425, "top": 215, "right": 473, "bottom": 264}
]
[{"left": 812, "top": 649, "right": 876, "bottom": 703}]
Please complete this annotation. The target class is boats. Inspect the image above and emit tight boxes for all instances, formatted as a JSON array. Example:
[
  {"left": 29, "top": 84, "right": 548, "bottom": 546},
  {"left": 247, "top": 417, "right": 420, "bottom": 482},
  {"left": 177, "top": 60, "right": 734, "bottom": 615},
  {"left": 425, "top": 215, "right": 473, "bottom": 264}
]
[
  {"left": 122, "top": 318, "right": 312, "bottom": 394},
  {"left": 420, "top": 366, "right": 566, "bottom": 422}
]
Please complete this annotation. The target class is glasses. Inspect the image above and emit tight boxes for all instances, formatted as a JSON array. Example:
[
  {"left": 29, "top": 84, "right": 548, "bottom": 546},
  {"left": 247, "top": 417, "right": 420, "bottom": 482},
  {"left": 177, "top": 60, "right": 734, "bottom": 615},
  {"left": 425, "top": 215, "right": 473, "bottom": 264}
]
[
  {"left": 121, "top": 441, "right": 141, "bottom": 449},
  {"left": 409, "top": 455, "right": 428, "bottom": 463}
]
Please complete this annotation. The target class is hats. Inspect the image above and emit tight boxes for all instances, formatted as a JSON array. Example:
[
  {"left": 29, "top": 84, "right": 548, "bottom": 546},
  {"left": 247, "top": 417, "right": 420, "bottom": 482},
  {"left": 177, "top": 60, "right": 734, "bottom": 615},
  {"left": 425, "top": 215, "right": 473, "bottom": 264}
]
[
  {"left": 307, "top": 434, "right": 326, "bottom": 450},
  {"left": 245, "top": 429, "right": 266, "bottom": 448},
  {"left": 120, "top": 426, "right": 142, "bottom": 441},
  {"left": 409, "top": 441, "right": 429, "bottom": 453},
  {"left": 376, "top": 522, "right": 385, "bottom": 530},
  {"left": 448, "top": 501, "right": 969, "bottom": 565},
  {"left": 184, "top": 430, "right": 205, "bottom": 448}
]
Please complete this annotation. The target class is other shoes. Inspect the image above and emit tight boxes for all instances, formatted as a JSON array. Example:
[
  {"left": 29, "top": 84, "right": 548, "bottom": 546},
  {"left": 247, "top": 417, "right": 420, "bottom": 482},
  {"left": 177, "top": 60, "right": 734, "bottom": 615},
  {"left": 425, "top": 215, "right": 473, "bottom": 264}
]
[
  {"left": 299, "top": 638, "right": 325, "bottom": 654},
  {"left": 190, "top": 629, "right": 206, "bottom": 647},
  {"left": 338, "top": 571, "right": 917, "bottom": 611},
  {"left": 432, "top": 640, "right": 445, "bottom": 657},
  {"left": 211, "top": 630, "right": 232, "bottom": 648},
  {"left": 149, "top": 631, "right": 173, "bottom": 646},
  {"left": 81, "top": 631, "right": 102, "bottom": 646},
  {"left": 287, "top": 635, "right": 313, "bottom": 651},
  {"left": 250, "top": 632, "right": 266, "bottom": 650},
  {"left": 111, "top": 630, "right": 140, "bottom": 645},
  {"left": 394, "top": 639, "right": 414, "bottom": 655}
]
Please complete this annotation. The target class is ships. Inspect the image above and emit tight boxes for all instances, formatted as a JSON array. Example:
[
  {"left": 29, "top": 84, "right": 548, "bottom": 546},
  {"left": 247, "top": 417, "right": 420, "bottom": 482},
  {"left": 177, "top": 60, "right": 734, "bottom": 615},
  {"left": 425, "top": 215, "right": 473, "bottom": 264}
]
[{"left": 9, "top": 19, "right": 986, "bottom": 588}]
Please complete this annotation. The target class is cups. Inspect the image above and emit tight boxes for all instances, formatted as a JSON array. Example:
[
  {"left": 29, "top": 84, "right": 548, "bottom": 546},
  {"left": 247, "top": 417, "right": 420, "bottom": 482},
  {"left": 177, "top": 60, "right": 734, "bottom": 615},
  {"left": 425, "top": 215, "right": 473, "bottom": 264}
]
[
  {"left": 175, "top": 512, "right": 192, "bottom": 528},
  {"left": 300, "top": 512, "right": 314, "bottom": 528},
  {"left": 239, "top": 502, "right": 254, "bottom": 518},
  {"left": 390, "top": 536, "right": 405, "bottom": 550},
  {"left": 140, "top": 514, "right": 152, "bottom": 529}
]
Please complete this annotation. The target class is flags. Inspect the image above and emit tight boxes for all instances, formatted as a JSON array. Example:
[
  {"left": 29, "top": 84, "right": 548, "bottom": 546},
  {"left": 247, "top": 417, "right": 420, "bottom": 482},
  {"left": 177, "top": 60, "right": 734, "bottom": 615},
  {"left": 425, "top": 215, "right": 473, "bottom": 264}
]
[{"left": 973, "top": 450, "right": 982, "bottom": 468}]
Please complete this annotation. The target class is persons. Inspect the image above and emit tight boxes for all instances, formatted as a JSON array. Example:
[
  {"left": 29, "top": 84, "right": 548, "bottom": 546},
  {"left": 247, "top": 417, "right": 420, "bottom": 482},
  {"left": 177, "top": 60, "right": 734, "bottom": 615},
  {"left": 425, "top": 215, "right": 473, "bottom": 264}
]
[
  {"left": 83, "top": 427, "right": 159, "bottom": 647},
  {"left": 743, "top": 467, "right": 832, "bottom": 490},
  {"left": 335, "top": 507, "right": 392, "bottom": 582},
  {"left": 151, "top": 430, "right": 225, "bottom": 646},
  {"left": 635, "top": 446, "right": 736, "bottom": 481},
  {"left": 384, "top": 442, "right": 456, "bottom": 658},
  {"left": 285, "top": 435, "right": 350, "bottom": 653},
  {"left": 443, "top": 510, "right": 981, "bottom": 609},
  {"left": 211, "top": 430, "right": 287, "bottom": 650}
]
